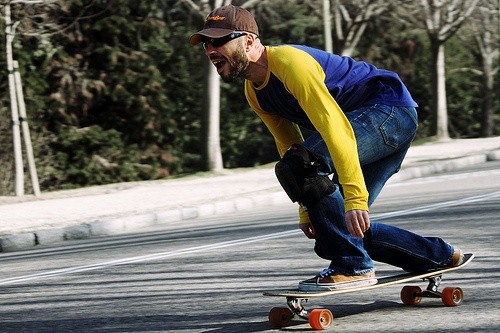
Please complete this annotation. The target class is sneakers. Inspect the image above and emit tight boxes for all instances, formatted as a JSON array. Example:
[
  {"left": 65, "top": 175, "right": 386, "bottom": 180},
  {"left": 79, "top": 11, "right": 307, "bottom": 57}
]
[
  {"left": 297, "top": 268, "right": 377, "bottom": 289},
  {"left": 450, "top": 248, "right": 464, "bottom": 266}
]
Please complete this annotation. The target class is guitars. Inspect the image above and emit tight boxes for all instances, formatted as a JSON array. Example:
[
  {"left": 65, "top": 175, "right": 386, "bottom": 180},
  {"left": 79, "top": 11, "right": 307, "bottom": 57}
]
[{"left": 261, "top": 252, "right": 476, "bottom": 330}]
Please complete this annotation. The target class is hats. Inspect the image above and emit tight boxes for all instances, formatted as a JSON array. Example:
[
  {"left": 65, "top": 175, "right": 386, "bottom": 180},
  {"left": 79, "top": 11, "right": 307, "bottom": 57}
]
[{"left": 189, "top": 5, "right": 260, "bottom": 44}]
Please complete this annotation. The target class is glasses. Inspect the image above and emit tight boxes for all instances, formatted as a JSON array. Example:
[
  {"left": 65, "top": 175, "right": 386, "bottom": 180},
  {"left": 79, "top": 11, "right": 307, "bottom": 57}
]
[{"left": 199, "top": 32, "right": 256, "bottom": 46}]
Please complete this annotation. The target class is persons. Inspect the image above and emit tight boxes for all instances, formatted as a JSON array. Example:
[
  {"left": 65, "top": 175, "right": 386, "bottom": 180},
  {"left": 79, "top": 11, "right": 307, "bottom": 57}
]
[{"left": 188, "top": 3, "right": 464, "bottom": 292}]
